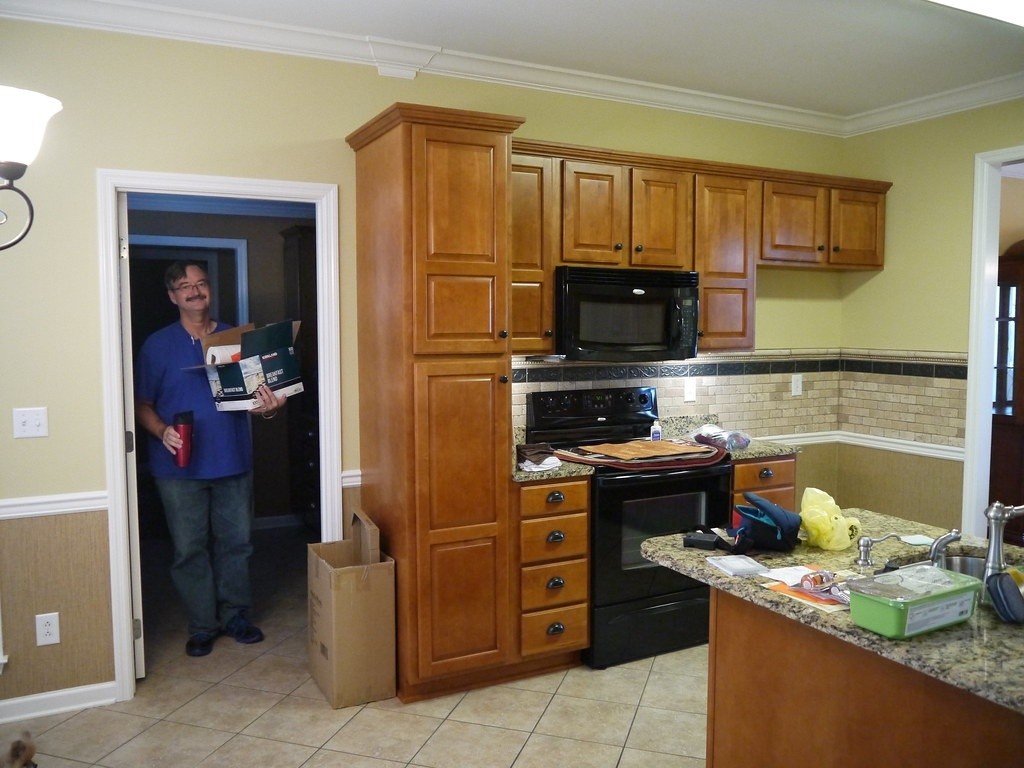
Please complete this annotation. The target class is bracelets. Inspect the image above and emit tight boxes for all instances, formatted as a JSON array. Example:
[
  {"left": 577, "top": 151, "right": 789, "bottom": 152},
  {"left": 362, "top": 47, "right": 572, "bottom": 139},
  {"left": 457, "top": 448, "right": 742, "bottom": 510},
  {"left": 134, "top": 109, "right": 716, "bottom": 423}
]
[{"left": 262, "top": 411, "right": 277, "bottom": 420}]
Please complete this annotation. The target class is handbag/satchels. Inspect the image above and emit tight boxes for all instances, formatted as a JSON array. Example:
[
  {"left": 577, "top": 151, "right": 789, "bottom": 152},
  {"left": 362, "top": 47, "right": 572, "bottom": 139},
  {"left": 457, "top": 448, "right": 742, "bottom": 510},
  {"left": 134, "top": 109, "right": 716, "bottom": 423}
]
[{"left": 725, "top": 490, "right": 801, "bottom": 552}]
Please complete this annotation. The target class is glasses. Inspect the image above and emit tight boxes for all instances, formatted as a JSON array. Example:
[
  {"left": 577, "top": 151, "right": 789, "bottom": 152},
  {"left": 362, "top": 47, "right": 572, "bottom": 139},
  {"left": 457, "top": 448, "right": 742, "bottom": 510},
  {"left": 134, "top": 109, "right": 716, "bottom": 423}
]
[{"left": 170, "top": 280, "right": 210, "bottom": 292}]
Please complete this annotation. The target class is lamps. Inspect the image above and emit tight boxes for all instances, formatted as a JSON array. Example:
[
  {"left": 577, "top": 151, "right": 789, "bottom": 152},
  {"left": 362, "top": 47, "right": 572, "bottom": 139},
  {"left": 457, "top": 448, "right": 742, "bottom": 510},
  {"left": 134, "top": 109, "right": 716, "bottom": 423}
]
[{"left": 0, "top": 85, "right": 63, "bottom": 250}]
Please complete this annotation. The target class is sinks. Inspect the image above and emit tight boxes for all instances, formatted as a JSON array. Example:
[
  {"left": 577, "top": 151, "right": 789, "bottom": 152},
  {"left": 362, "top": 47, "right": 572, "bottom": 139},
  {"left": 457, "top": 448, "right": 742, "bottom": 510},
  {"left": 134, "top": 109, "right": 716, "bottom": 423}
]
[{"left": 886, "top": 545, "right": 1024, "bottom": 585}]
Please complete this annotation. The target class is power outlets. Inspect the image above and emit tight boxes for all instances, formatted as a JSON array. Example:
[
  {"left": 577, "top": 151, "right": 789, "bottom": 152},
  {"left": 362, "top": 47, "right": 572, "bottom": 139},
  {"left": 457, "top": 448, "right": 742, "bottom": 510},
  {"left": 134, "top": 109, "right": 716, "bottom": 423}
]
[
  {"left": 34, "top": 612, "right": 61, "bottom": 645},
  {"left": 792, "top": 375, "right": 802, "bottom": 396},
  {"left": 684, "top": 382, "right": 696, "bottom": 402}
]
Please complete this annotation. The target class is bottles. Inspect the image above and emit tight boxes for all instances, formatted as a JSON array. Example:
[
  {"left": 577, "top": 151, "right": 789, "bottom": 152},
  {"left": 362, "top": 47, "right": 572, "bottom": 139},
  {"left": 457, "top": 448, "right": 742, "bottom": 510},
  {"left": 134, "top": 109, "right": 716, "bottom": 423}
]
[
  {"left": 651, "top": 421, "right": 661, "bottom": 441},
  {"left": 174, "top": 410, "right": 193, "bottom": 467},
  {"left": 801, "top": 569, "right": 837, "bottom": 590}
]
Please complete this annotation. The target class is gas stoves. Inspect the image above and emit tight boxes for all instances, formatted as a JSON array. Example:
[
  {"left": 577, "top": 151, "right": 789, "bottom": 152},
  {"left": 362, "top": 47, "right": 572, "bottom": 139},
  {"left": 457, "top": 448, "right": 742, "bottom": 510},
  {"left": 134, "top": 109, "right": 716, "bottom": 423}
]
[{"left": 525, "top": 386, "right": 731, "bottom": 475}]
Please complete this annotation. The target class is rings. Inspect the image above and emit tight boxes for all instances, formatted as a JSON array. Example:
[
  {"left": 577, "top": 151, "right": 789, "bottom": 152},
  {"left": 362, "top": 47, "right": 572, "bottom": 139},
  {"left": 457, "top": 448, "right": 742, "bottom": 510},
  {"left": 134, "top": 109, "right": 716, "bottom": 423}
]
[{"left": 263, "top": 407, "right": 267, "bottom": 409}]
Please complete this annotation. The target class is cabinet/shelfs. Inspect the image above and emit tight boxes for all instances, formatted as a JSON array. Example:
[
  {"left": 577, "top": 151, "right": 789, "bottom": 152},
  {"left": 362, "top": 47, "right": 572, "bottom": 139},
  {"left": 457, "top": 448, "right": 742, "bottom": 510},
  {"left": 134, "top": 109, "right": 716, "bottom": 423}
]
[
  {"left": 560, "top": 143, "right": 693, "bottom": 272},
  {"left": 695, "top": 158, "right": 757, "bottom": 349},
  {"left": 395, "top": 101, "right": 511, "bottom": 355},
  {"left": 762, "top": 167, "right": 894, "bottom": 269},
  {"left": 511, "top": 137, "right": 562, "bottom": 354},
  {"left": 734, "top": 454, "right": 798, "bottom": 528},
  {"left": 519, "top": 476, "right": 590, "bottom": 681},
  {"left": 409, "top": 358, "right": 512, "bottom": 703}
]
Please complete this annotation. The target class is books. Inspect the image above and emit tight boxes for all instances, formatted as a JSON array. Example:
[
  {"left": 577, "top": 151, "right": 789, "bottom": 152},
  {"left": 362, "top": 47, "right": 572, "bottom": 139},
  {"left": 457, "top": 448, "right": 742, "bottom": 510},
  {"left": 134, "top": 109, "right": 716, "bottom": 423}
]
[{"left": 706, "top": 554, "right": 769, "bottom": 576}]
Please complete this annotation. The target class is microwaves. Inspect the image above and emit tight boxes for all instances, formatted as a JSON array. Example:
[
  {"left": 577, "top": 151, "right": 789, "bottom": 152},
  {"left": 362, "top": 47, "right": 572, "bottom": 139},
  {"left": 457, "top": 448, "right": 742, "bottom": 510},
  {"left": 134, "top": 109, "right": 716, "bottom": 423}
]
[{"left": 555, "top": 265, "right": 699, "bottom": 363}]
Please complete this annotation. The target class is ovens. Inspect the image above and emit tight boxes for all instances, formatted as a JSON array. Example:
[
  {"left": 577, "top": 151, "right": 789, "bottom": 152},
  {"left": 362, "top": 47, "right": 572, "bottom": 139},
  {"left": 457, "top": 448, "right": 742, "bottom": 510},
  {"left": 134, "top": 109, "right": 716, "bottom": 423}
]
[{"left": 581, "top": 458, "right": 734, "bottom": 669}]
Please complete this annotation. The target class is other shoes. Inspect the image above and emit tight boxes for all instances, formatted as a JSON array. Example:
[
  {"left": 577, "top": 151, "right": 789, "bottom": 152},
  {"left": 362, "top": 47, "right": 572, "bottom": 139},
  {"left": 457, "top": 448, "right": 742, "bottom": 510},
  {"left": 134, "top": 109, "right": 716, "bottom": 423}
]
[
  {"left": 223, "top": 613, "right": 263, "bottom": 644},
  {"left": 186, "top": 632, "right": 216, "bottom": 658}
]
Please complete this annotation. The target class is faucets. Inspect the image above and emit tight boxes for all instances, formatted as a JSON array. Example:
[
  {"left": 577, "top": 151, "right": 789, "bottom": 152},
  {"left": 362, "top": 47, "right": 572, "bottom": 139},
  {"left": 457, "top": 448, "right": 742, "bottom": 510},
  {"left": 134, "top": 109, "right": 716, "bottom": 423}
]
[{"left": 929, "top": 528, "right": 962, "bottom": 570}]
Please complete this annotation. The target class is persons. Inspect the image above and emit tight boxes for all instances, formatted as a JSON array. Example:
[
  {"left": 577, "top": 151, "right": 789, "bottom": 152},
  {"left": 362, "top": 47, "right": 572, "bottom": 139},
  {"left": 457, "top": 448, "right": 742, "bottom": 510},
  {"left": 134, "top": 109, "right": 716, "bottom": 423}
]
[{"left": 133, "top": 259, "right": 287, "bottom": 656}]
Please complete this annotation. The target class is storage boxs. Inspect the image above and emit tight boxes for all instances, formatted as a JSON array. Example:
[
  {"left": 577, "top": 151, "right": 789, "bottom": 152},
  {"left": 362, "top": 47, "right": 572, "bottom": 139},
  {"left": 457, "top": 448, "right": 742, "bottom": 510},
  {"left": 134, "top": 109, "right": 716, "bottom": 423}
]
[
  {"left": 178, "top": 317, "right": 302, "bottom": 413},
  {"left": 307, "top": 508, "right": 396, "bottom": 711},
  {"left": 851, "top": 567, "right": 981, "bottom": 638}
]
